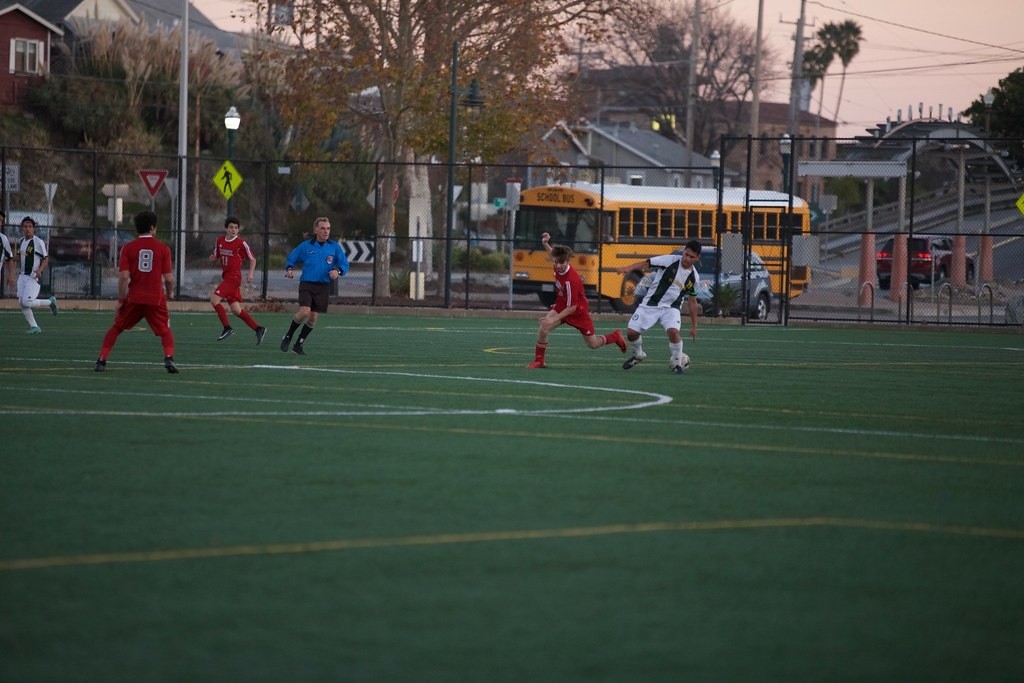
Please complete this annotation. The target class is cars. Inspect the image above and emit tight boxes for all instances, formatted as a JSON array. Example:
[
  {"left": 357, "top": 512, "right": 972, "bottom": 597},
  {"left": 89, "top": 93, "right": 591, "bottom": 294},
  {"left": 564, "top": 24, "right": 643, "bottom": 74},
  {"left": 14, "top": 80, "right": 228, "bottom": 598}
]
[
  {"left": 632, "top": 246, "right": 774, "bottom": 323},
  {"left": 875, "top": 234, "right": 976, "bottom": 290},
  {"left": 43, "top": 226, "right": 135, "bottom": 265}
]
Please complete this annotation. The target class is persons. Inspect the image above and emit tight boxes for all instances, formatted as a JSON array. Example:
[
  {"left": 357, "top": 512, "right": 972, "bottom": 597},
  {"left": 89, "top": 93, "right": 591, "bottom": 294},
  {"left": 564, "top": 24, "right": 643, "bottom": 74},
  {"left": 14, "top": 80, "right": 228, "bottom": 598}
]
[
  {"left": 209, "top": 217, "right": 267, "bottom": 347},
  {"left": 95, "top": 212, "right": 179, "bottom": 374},
  {"left": 525, "top": 232, "right": 626, "bottom": 368},
  {"left": 617, "top": 240, "right": 702, "bottom": 375},
  {"left": 0, "top": 210, "right": 16, "bottom": 289},
  {"left": 280, "top": 218, "right": 350, "bottom": 355},
  {"left": 15, "top": 216, "right": 57, "bottom": 333}
]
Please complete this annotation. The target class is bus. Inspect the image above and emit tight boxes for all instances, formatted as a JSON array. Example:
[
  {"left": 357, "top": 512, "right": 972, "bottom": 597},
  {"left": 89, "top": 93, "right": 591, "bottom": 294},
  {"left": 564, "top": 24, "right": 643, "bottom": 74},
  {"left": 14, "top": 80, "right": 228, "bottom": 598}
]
[{"left": 509, "top": 182, "right": 813, "bottom": 314}]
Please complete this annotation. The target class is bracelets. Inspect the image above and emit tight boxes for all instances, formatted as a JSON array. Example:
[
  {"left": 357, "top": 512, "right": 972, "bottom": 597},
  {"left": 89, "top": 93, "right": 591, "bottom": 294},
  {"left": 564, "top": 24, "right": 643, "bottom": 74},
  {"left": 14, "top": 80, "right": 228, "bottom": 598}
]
[
  {"left": 287, "top": 268, "right": 293, "bottom": 271},
  {"left": 337, "top": 269, "right": 341, "bottom": 275},
  {"left": 39, "top": 267, "right": 43, "bottom": 272},
  {"left": 118, "top": 297, "right": 125, "bottom": 303}
]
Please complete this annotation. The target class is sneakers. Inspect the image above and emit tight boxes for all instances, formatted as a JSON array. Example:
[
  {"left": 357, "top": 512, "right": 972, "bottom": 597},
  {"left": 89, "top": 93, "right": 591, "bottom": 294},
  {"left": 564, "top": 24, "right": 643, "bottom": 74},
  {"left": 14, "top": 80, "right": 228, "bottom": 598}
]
[
  {"left": 674, "top": 365, "right": 683, "bottom": 374},
  {"left": 255, "top": 327, "right": 267, "bottom": 347},
  {"left": 94, "top": 360, "right": 106, "bottom": 371},
  {"left": 165, "top": 357, "right": 179, "bottom": 373},
  {"left": 293, "top": 342, "right": 306, "bottom": 355},
  {"left": 49, "top": 296, "right": 57, "bottom": 315},
  {"left": 614, "top": 329, "right": 627, "bottom": 353},
  {"left": 217, "top": 328, "right": 234, "bottom": 340},
  {"left": 623, "top": 352, "right": 647, "bottom": 369},
  {"left": 281, "top": 335, "right": 292, "bottom": 352},
  {"left": 26, "top": 327, "right": 41, "bottom": 334},
  {"left": 525, "top": 362, "right": 547, "bottom": 368}
]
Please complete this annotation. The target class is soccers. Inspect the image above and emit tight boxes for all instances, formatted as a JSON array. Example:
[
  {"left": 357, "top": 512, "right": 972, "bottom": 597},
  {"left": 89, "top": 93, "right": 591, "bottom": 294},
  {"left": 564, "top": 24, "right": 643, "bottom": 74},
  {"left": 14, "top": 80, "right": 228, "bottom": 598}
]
[{"left": 669, "top": 352, "right": 691, "bottom": 373}]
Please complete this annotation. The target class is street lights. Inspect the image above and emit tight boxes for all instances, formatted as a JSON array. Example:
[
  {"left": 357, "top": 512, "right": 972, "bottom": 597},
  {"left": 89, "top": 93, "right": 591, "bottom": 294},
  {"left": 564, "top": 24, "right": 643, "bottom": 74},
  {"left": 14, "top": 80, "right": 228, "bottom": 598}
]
[
  {"left": 444, "top": 39, "right": 487, "bottom": 308},
  {"left": 710, "top": 149, "right": 720, "bottom": 188},
  {"left": 779, "top": 132, "right": 792, "bottom": 194},
  {"left": 222, "top": 105, "right": 242, "bottom": 218}
]
